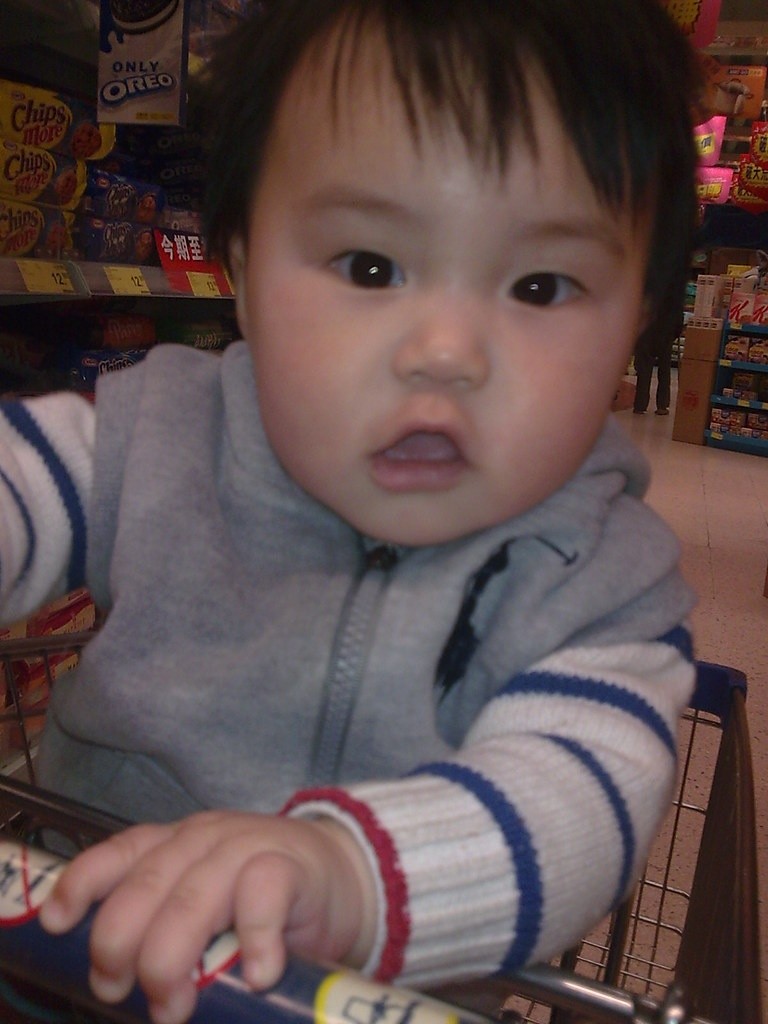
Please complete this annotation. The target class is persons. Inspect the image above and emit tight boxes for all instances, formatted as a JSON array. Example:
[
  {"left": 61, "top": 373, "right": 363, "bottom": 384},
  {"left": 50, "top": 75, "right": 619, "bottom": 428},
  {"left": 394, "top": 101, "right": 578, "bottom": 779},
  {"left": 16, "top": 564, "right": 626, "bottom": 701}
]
[
  {"left": 0, "top": 0, "right": 697, "bottom": 1023},
  {"left": 631, "top": 301, "right": 684, "bottom": 414}
]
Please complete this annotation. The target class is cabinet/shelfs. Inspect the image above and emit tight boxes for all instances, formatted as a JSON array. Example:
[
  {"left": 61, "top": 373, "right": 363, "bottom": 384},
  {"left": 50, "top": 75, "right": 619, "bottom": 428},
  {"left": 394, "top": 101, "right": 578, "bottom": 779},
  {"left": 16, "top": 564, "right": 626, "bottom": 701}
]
[{"left": 703, "top": 322, "right": 768, "bottom": 458}]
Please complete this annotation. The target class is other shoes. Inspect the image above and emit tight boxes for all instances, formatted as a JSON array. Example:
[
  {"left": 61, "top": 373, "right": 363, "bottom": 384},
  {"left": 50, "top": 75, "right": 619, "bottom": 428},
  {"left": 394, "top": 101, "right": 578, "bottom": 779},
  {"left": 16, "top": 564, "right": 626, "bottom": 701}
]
[
  {"left": 632, "top": 408, "right": 645, "bottom": 414},
  {"left": 655, "top": 408, "right": 669, "bottom": 415}
]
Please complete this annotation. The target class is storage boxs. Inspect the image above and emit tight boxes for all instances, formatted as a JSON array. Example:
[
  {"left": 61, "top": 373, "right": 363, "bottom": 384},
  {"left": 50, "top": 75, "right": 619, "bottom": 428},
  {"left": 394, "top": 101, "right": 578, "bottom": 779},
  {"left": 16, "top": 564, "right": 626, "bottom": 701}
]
[
  {"left": 694, "top": 264, "right": 768, "bottom": 327},
  {"left": 710, "top": 334, "right": 768, "bottom": 440},
  {"left": 672, "top": 359, "right": 717, "bottom": 446},
  {"left": 0, "top": 0, "right": 249, "bottom": 754},
  {"left": 683, "top": 325, "right": 722, "bottom": 363}
]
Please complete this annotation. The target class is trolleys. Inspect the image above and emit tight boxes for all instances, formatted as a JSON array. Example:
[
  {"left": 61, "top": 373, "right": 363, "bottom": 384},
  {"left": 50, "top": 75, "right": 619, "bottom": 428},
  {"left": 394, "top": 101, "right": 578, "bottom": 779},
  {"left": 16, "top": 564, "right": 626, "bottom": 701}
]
[{"left": 0, "top": 627, "right": 758, "bottom": 1024}]
